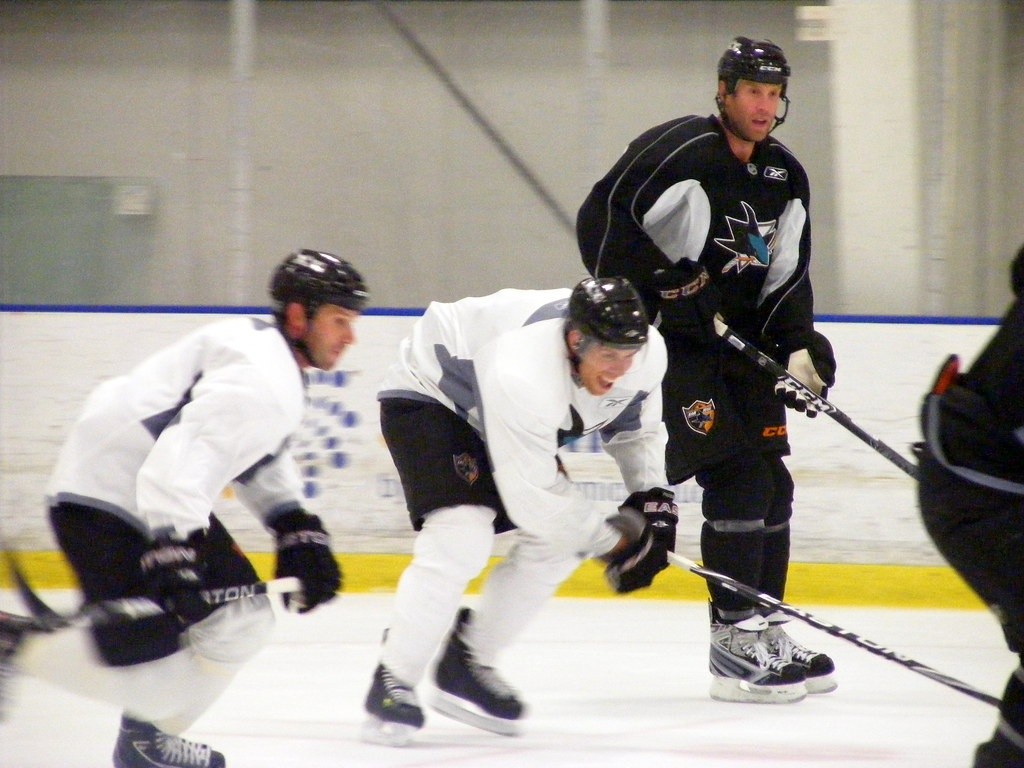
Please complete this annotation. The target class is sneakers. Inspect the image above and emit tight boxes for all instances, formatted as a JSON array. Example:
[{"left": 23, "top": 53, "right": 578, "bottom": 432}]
[
  {"left": 419, "top": 630, "right": 525, "bottom": 736},
  {"left": 0, "top": 610, "right": 29, "bottom": 671},
  {"left": 708, "top": 597, "right": 807, "bottom": 704},
  {"left": 360, "top": 664, "right": 423, "bottom": 747},
  {"left": 112, "top": 715, "right": 225, "bottom": 768},
  {"left": 757, "top": 612, "right": 838, "bottom": 695}
]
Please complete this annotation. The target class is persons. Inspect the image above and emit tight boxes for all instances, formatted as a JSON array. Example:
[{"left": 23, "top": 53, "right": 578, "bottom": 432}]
[
  {"left": 576, "top": 36, "right": 842, "bottom": 702},
  {"left": 0, "top": 248, "right": 372, "bottom": 768},
  {"left": 920, "top": 247, "right": 1024, "bottom": 768},
  {"left": 361, "top": 275, "right": 678, "bottom": 748}
]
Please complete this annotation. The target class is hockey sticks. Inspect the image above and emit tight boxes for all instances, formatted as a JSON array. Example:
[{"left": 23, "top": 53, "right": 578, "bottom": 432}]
[
  {"left": 4, "top": 547, "right": 304, "bottom": 630},
  {"left": 712, "top": 317, "right": 923, "bottom": 480},
  {"left": 665, "top": 550, "right": 1003, "bottom": 713}
]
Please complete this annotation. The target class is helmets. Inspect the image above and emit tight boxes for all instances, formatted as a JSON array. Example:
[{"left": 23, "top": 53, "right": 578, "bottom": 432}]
[
  {"left": 569, "top": 276, "right": 648, "bottom": 345},
  {"left": 718, "top": 36, "right": 792, "bottom": 99},
  {"left": 268, "top": 249, "right": 370, "bottom": 319}
]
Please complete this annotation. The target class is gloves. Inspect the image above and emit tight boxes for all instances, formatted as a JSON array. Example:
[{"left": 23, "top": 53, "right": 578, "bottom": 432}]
[
  {"left": 655, "top": 258, "right": 720, "bottom": 356},
  {"left": 264, "top": 509, "right": 342, "bottom": 615},
  {"left": 775, "top": 332, "right": 837, "bottom": 419},
  {"left": 604, "top": 486, "right": 678, "bottom": 594},
  {"left": 139, "top": 539, "right": 210, "bottom": 632}
]
